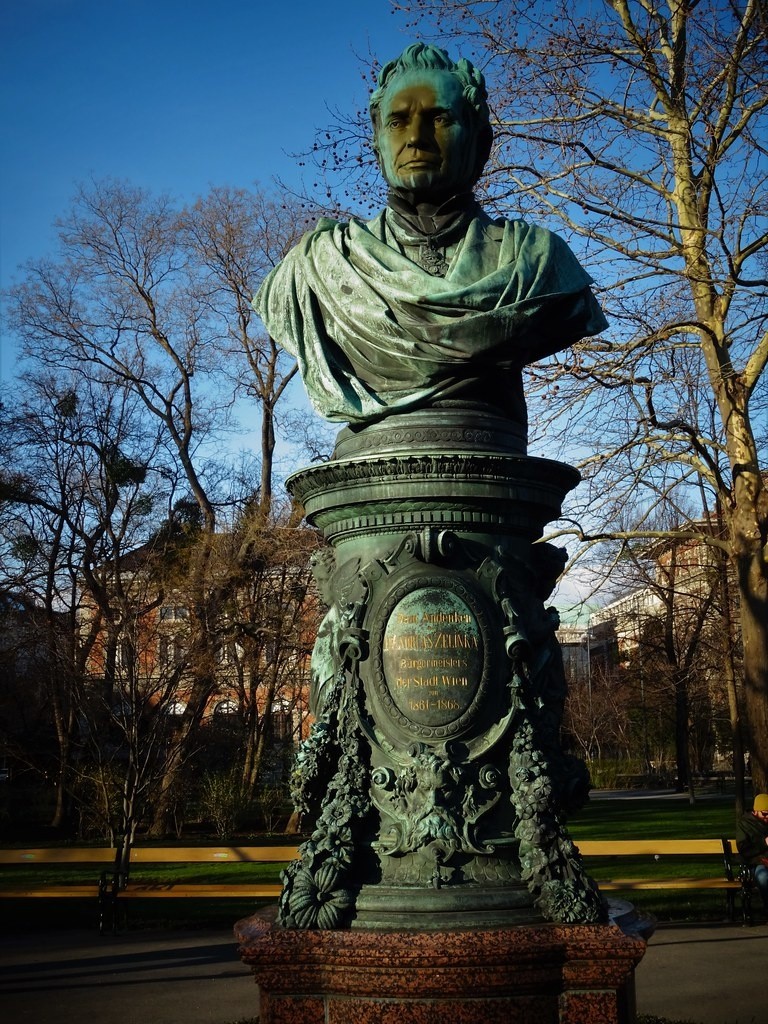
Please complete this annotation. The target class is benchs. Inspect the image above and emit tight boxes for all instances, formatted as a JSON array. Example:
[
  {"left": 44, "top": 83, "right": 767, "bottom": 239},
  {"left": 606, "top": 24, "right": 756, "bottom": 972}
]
[
  {"left": 0, "top": 838, "right": 750, "bottom": 937},
  {"left": 685, "top": 771, "right": 726, "bottom": 794}
]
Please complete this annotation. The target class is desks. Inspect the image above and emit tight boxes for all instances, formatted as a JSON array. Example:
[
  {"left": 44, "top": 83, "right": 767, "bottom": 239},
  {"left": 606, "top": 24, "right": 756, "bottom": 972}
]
[{"left": 613, "top": 774, "right": 644, "bottom": 790}]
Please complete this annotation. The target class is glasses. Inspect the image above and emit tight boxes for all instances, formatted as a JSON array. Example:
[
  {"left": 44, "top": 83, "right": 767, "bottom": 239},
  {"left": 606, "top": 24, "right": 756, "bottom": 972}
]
[{"left": 760, "top": 811, "right": 768, "bottom": 819}]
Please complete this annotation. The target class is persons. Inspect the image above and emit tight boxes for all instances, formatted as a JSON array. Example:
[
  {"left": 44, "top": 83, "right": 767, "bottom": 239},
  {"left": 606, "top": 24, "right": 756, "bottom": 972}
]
[
  {"left": 250, "top": 44, "right": 609, "bottom": 426},
  {"left": 736, "top": 792, "right": 768, "bottom": 910}
]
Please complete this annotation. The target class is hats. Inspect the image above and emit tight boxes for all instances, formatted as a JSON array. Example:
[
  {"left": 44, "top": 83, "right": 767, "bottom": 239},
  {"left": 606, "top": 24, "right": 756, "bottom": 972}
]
[{"left": 753, "top": 794, "right": 768, "bottom": 811}]
